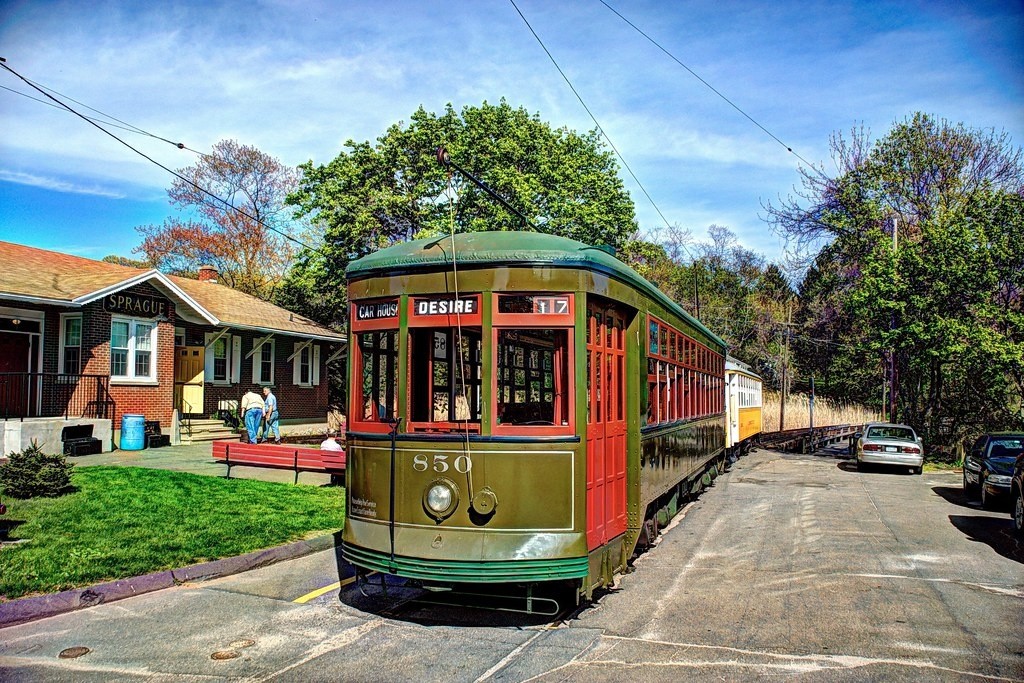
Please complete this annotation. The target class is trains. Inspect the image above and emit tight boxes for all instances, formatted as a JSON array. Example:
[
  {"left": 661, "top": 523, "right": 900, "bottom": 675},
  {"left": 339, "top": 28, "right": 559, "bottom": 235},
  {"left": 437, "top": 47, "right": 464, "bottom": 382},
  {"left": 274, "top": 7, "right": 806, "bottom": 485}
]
[{"left": 338, "top": 229, "right": 763, "bottom": 615}]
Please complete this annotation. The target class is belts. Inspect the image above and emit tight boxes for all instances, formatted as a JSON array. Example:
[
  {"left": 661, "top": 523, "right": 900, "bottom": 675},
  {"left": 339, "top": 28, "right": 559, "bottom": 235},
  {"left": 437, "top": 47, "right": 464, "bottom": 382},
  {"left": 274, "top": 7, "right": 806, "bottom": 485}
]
[
  {"left": 266, "top": 410, "right": 276, "bottom": 413},
  {"left": 246, "top": 407, "right": 261, "bottom": 411}
]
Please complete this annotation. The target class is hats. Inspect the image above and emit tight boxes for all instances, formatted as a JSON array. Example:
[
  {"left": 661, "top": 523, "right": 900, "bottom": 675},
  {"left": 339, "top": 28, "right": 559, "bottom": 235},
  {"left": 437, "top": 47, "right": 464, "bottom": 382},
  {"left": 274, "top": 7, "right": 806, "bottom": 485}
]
[{"left": 328, "top": 428, "right": 337, "bottom": 434}]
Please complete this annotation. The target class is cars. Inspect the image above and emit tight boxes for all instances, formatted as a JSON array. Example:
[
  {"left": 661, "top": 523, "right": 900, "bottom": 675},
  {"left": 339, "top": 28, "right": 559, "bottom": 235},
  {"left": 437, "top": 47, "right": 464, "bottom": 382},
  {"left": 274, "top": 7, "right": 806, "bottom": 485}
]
[
  {"left": 963, "top": 431, "right": 1024, "bottom": 507},
  {"left": 854, "top": 425, "right": 924, "bottom": 474},
  {"left": 1009, "top": 451, "right": 1024, "bottom": 532}
]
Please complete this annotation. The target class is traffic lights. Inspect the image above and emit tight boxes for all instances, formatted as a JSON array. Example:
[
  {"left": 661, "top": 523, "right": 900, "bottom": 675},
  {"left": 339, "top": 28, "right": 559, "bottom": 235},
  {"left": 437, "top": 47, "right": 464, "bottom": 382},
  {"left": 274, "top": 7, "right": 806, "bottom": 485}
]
[{"left": 809, "top": 377, "right": 815, "bottom": 400}]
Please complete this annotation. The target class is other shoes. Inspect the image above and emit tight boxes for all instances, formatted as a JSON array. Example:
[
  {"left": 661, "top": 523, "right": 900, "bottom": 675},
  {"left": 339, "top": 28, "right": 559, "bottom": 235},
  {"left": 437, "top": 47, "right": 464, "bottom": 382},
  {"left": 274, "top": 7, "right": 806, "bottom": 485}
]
[
  {"left": 271, "top": 439, "right": 281, "bottom": 445},
  {"left": 262, "top": 441, "right": 268, "bottom": 444}
]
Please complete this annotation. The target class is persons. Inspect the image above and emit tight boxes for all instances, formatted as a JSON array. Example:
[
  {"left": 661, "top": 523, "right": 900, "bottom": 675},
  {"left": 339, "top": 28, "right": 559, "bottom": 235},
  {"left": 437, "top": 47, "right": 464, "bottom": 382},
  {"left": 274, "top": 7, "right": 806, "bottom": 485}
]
[
  {"left": 260, "top": 386, "right": 280, "bottom": 443},
  {"left": 241, "top": 388, "right": 266, "bottom": 444},
  {"left": 320, "top": 429, "right": 342, "bottom": 483}
]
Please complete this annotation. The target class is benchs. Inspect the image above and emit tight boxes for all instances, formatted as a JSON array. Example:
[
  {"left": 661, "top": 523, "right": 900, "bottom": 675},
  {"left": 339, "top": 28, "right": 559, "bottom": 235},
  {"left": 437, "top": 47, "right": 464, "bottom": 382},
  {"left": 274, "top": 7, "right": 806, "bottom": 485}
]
[{"left": 212, "top": 440, "right": 345, "bottom": 485}]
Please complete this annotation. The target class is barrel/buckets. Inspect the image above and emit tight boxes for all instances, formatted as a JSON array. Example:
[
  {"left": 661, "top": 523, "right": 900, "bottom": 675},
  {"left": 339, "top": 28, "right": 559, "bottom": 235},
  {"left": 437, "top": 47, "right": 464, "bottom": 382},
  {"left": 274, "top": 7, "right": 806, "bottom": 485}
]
[{"left": 120, "top": 414, "right": 145, "bottom": 451}]
